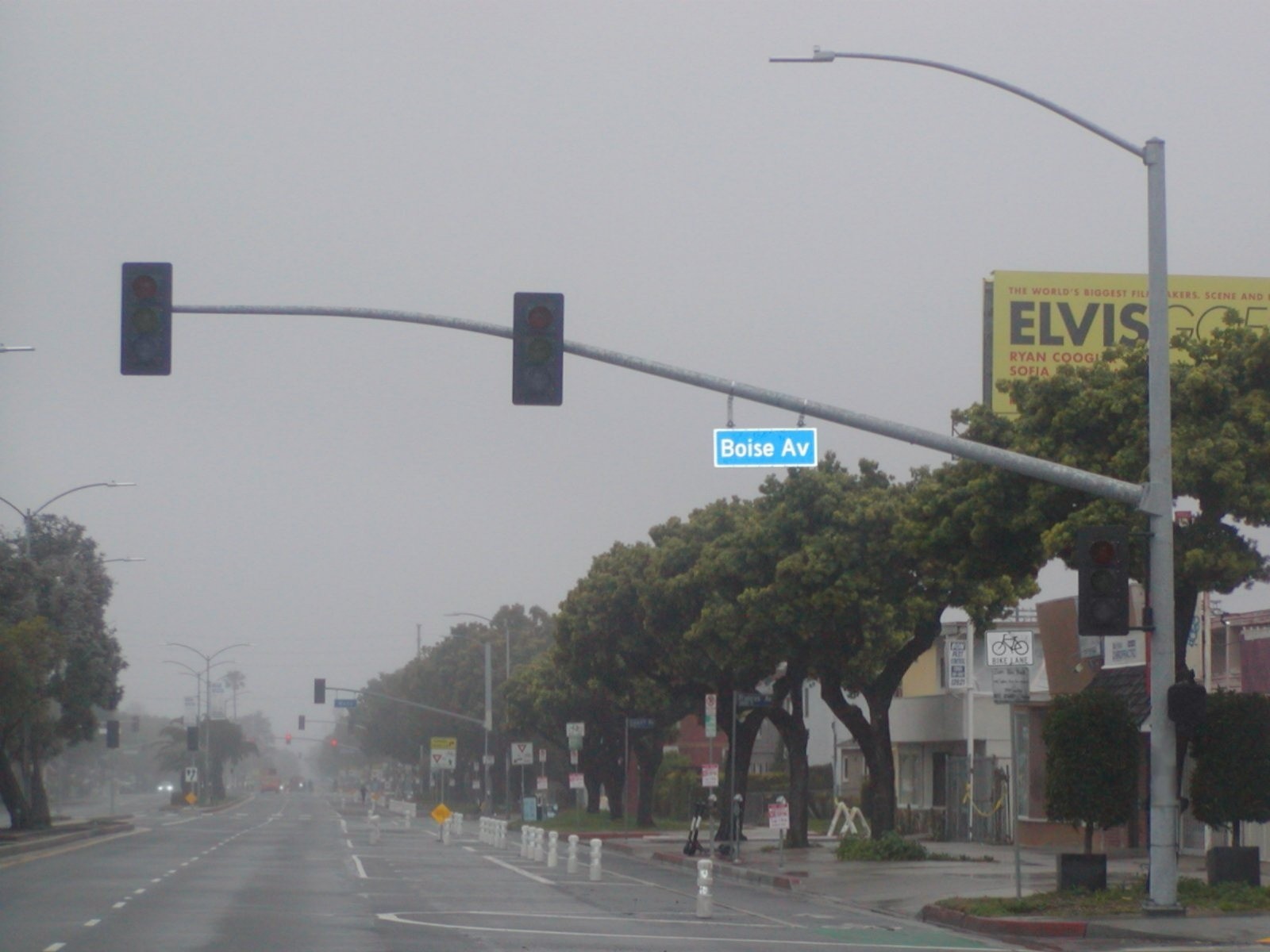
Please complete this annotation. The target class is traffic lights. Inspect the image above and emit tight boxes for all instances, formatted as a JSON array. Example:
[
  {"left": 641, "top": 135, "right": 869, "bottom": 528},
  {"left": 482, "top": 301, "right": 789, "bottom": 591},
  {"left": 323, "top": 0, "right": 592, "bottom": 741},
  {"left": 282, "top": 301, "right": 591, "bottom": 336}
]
[
  {"left": 1073, "top": 523, "right": 1133, "bottom": 636},
  {"left": 298, "top": 715, "right": 305, "bottom": 731},
  {"left": 285, "top": 732, "right": 292, "bottom": 745},
  {"left": 312, "top": 678, "right": 327, "bottom": 705},
  {"left": 117, "top": 260, "right": 173, "bottom": 377},
  {"left": 187, "top": 725, "right": 200, "bottom": 751},
  {"left": 331, "top": 739, "right": 337, "bottom": 753},
  {"left": 105, "top": 720, "right": 119, "bottom": 750},
  {"left": 509, "top": 291, "right": 566, "bottom": 407}
]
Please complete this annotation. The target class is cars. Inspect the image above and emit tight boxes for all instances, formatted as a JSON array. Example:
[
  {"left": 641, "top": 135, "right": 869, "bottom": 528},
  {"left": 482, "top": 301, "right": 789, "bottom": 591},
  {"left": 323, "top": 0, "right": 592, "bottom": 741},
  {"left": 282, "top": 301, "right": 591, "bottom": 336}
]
[{"left": 157, "top": 780, "right": 174, "bottom": 794}]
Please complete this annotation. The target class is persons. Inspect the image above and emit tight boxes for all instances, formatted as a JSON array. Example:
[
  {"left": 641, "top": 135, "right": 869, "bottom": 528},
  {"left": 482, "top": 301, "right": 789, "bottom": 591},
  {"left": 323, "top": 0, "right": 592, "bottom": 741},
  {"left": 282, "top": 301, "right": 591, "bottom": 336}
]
[{"left": 360, "top": 784, "right": 367, "bottom": 800}]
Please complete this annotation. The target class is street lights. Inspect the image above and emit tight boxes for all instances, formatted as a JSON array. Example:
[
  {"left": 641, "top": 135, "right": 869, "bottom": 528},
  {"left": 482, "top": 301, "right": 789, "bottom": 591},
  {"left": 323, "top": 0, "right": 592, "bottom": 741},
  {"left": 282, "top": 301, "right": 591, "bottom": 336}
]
[
  {"left": 0, "top": 344, "right": 36, "bottom": 353},
  {"left": 441, "top": 612, "right": 513, "bottom": 817},
  {"left": 0, "top": 481, "right": 148, "bottom": 568},
  {"left": 768, "top": 44, "right": 1186, "bottom": 909},
  {"left": 162, "top": 641, "right": 251, "bottom": 805}
]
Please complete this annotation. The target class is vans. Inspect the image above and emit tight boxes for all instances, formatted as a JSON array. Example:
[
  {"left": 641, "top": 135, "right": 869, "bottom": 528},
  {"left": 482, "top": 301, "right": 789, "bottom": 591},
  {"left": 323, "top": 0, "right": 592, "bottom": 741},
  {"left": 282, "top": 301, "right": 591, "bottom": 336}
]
[{"left": 289, "top": 775, "right": 306, "bottom": 793}]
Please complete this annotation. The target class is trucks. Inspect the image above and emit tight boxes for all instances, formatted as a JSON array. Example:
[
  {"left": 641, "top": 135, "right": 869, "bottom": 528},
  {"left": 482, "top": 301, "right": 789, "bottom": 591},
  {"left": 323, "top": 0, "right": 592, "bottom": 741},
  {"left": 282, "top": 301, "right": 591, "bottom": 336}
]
[{"left": 258, "top": 767, "right": 281, "bottom": 795}]
